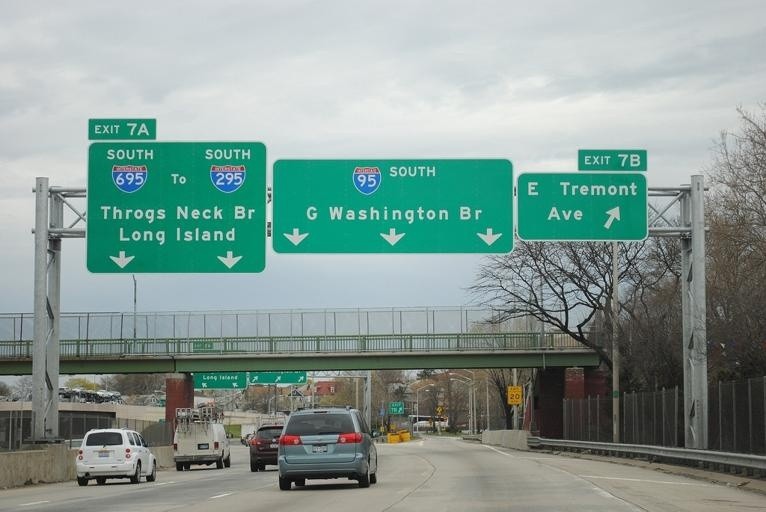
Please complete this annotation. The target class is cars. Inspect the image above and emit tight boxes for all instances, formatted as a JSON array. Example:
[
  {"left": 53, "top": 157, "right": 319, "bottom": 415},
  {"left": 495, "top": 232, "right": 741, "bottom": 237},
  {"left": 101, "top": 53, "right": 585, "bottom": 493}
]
[
  {"left": 371, "top": 421, "right": 408, "bottom": 438},
  {"left": 75, "top": 428, "right": 157, "bottom": 486},
  {"left": 0, "top": 385, "right": 123, "bottom": 404}
]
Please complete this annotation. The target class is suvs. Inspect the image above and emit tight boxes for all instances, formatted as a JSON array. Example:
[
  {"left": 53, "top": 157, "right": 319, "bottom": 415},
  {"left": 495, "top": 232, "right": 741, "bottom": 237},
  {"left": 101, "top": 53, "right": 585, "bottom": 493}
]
[
  {"left": 249, "top": 422, "right": 284, "bottom": 472},
  {"left": 277, "top": 404, "right": 378, "bottom": 490}
]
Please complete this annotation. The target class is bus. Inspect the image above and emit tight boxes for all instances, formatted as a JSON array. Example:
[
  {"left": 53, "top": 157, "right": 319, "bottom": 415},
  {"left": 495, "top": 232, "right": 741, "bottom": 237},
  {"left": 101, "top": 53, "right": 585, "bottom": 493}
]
[{"left": 407, "top": 415, "right": 449, "bottom": 431}]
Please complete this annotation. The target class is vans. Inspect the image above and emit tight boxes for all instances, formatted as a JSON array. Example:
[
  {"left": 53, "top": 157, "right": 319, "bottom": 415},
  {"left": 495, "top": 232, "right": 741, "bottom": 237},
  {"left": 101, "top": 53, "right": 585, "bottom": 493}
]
[{"left": 172, "top": 419, "right": 233, "bottom": 471}]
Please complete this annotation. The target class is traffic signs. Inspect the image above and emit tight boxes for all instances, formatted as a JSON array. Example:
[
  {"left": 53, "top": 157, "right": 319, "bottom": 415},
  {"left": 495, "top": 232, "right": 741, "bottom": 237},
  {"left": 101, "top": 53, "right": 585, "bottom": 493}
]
[
  {"left": 385, "top": 402, "right": 404, "bottom": 415},
  {"left": 193, "top": 371, "right": 248, "bottom": 390},
  {"left": 87, "top": 141, "right": 266, "bottom": 273},
  {"left": 192, "top": 341, "right": 214, "bottom": 353},
  {"left": 516, "top": 172, "right": 649, "bottom": 242},
  {"left": 249, "top": 370, "right": 308, "bottom": 384},
  {"left": 271, "top": 159, "right": 514, "bottom": 255}
]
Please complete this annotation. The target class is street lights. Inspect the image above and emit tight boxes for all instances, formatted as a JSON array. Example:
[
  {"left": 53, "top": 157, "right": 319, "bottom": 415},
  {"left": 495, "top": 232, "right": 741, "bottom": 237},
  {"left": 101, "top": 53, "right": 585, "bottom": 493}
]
[
  {"left": 417, "top": 384, "right": 435, "bottom": 432},
  {"left": 449, "top": 369, "right": 476, "bottom": 435}
]
